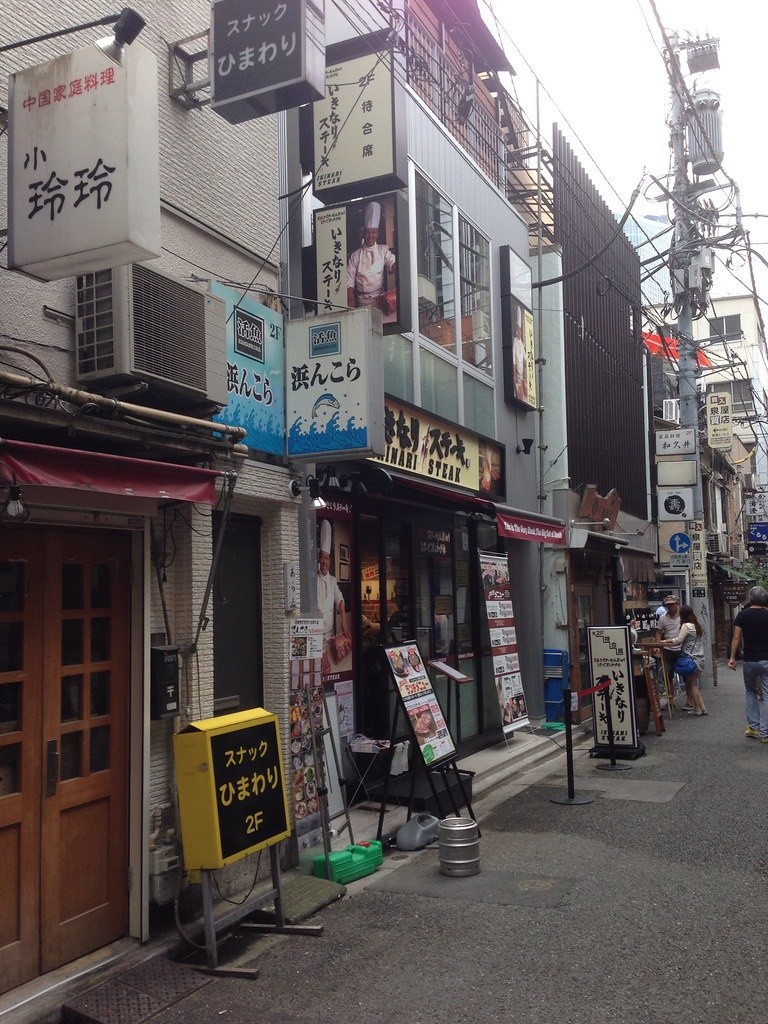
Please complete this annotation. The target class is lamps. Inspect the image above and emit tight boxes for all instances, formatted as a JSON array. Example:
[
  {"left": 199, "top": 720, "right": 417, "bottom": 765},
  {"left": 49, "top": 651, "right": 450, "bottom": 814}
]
[
  {"left": 516, "top": 438, "right": 533, "bottom": 454},
  {"left": 571, "top": 517, "right": 610, "bottom": 528},
  {"left": 0, "top": 7, "right": 146, "bottom": 68},
  {"left": 0, "top": 461, "right": 32, "bottom": 526},
  {"left": 610, "top": 529, "right": 644, "bottom": 536},
  {"left": 290, "top": 466, "right": 366, "bottom": 510}
]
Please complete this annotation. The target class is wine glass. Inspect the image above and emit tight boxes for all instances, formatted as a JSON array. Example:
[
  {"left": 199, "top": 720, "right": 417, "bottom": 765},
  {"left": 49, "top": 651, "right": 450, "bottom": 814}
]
[{"left": 366, "top": 586, "right": 371, "bottom": 603}]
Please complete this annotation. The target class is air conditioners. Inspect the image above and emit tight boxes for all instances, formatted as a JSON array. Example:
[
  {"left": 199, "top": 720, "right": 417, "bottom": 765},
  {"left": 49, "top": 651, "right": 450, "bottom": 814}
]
[
  {"left": 74, "top": 262, "right": 229, "bottom": 408},
  {"left": 733, "top": 543, "right": 745, "bottom": 563},
  {"left": 663, "top": 399, "right": 680, "bottom": 424},
  {"left": 708, "top": 532, "right": 730, "bottom": 554}
]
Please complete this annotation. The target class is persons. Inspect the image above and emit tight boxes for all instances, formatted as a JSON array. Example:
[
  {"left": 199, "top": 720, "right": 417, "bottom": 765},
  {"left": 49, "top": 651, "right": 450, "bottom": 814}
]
[
  {"left": 727, "top": 586, "right": 768, "bottom": 743},
  {"left": 513, "top": 305, "right": 527, "bottom": 398},
  {"left": 392, "top": 594, "right": 409, "bottom": 621},
  {"left": 656, "top": 601, "right": 668, "bottom": 614},
  {"left": 317, "top": 519, "right": 350, "bottom": 638},
  {"left": 348, "top": 202, "right": 396, "bottom": 315},
  {"left": 659, "top": 605, "right": 708, "bottom": 715},
  {"left": 655, "top": 595, "right": 694, "bottom": 711}
]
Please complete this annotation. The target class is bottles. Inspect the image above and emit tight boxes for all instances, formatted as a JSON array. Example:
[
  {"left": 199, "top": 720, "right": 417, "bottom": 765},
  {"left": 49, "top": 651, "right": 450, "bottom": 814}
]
[{"left": 626, "top": 609, "right": 662, "bottom": 632}]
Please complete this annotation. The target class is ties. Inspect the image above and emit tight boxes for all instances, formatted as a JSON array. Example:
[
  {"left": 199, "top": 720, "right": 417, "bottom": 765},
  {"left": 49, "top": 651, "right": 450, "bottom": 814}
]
[
  {"left": 319, "top": 574, "right": 328, "bottom": 598},
  {"left": 363, "top": 247, "right": 375, "bottom": 264}
]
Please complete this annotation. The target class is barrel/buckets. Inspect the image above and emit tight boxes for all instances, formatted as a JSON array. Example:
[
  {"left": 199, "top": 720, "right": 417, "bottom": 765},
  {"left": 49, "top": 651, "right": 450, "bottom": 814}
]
[
  {"left": 438, "top": 818, "right": 482, "bottom": 878},
  {"left": 312, "top": 840, "right": 384, "bottom": 885}
]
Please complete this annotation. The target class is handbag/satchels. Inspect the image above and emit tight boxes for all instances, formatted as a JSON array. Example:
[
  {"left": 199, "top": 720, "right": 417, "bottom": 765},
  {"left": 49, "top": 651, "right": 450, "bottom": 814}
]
[{"left": 675, "top": 657, "right": 698, "bottom": 675}]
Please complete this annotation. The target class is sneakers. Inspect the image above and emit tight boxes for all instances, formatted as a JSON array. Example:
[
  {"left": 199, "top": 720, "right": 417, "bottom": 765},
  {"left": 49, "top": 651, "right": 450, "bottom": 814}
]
[
  {"left": 746, "top": 727, "right": 759, "bottom": 736},
  {"left": 761, "top": 735, "right": 768, "bottom": 742}
]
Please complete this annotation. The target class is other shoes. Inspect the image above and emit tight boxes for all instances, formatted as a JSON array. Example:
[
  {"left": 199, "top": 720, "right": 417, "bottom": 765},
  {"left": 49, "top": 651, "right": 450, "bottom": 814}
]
[
  {"left": 756, "top": 691, "right": 764, "bottom": 700},
  {"left": 681, "top": 703, "right": 695, "bottom": 710},
  {"left": 688, "top": 708, "right": 702, "bottom": 716},
  {"left": 701, "top": 708, "right": 708, "bottom": 715}
]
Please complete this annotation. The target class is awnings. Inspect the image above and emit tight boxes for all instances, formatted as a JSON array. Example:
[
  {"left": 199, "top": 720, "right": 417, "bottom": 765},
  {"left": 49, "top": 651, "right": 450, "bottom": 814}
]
[
  {"left": 0, "top": 440, "right": 237, "bottom": 648},
  {"left": 363, "top": 470, "right": 567, "bottom": 544},
  {"left": 615, "top": 544, "right": 656, "bottom": 582}
]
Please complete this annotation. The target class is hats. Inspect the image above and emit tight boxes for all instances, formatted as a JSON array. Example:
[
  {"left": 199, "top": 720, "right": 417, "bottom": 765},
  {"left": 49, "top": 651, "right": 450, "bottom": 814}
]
[
  {"left": 363, "top": 201, "right": 381, "bottom": 227},
  {"left": 664, "top": 595, "right": 679, "bottom": 604},
  {"left": 320, "top": 519, "right": 331, "bottom": 555}
]
